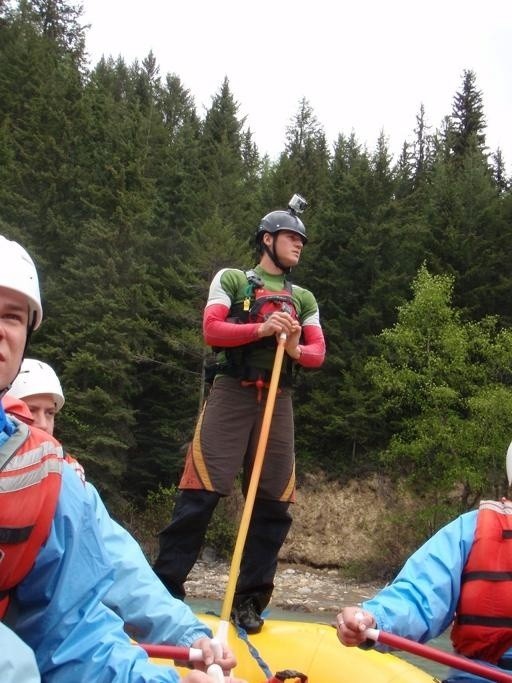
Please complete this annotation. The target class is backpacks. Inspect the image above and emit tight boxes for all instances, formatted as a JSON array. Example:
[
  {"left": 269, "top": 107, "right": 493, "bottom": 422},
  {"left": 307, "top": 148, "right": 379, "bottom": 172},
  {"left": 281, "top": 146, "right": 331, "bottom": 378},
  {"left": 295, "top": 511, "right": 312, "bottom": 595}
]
[{"left": 204, "top": 267, "right": 301, "bottom": 386}]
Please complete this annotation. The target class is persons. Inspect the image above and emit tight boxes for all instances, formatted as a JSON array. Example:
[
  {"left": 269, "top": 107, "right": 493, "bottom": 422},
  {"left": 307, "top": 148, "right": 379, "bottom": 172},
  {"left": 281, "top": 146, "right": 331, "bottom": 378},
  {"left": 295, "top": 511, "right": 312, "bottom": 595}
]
[
  {"left": 0, "top": 233, "right": 247, "bottom": 683},
  {"left": 337, "top": 440, "right": 512, "bottom": 683},
  {"left": 152, "top": 211, "right": 327, "bottom": 634},
  {"left": 1, "top": 358, "right": 236, "bottom": 676}
]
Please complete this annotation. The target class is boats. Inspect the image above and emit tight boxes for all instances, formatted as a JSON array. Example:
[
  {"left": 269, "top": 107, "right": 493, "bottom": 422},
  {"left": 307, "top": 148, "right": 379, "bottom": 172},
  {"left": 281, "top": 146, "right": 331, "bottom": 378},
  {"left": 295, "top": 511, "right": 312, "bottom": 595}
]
[{"left": 129, "top": 610, "right": 443, "bottom": 683}]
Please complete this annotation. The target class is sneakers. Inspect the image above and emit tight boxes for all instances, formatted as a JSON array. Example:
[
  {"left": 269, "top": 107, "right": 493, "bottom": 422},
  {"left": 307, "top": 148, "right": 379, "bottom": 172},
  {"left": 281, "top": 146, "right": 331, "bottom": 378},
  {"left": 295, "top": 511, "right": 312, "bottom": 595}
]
[{"left": 231, "top": 600, "right": 266, "bottom": 635}]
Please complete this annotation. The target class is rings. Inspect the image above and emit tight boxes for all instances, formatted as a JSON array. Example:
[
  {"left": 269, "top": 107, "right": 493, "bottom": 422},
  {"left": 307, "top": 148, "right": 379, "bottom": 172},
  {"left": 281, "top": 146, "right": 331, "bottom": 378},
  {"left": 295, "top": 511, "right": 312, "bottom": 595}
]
[{"left": 338, "top": 622, "right": 345, "bottom": 629}]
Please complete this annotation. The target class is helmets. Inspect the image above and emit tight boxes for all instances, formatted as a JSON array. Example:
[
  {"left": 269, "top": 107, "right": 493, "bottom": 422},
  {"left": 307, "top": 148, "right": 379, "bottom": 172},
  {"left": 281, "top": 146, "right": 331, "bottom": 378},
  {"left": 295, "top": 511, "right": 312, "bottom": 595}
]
[
  {"left": 0, "top": 235, "right": 45, "bottom": 332},
  {"left": 256, "top": 209, "right": 309, "bottom": 246},
  {"left": 5, "top": 357, "right": 66, "bottom": 414}
]
